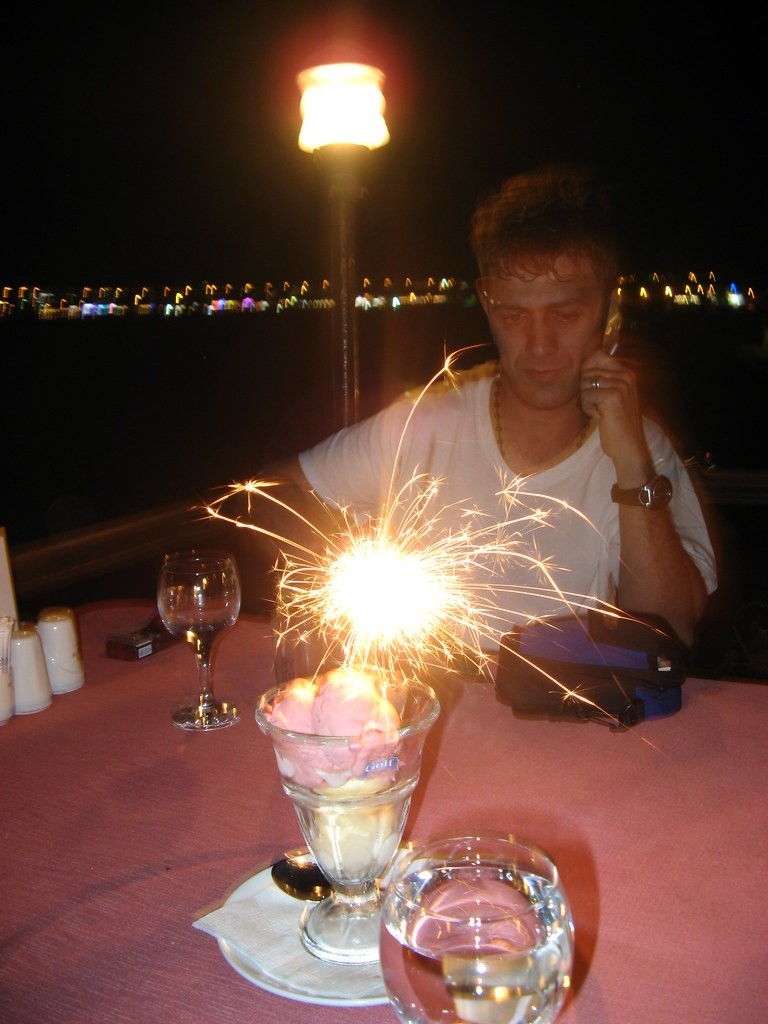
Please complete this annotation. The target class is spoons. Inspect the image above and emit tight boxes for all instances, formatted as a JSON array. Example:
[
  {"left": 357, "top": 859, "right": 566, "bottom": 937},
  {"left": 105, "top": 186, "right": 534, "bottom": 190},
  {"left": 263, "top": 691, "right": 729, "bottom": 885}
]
[{"left": 271, "top": 859, "right": 389, "bottom": 901}]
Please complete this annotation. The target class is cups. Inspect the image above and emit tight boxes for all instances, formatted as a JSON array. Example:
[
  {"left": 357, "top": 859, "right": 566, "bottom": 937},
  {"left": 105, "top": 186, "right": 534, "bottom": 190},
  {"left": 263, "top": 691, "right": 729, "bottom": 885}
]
[
  {"left": 10, "top": 630, "right": 53, "bottom": 716},
  {"left": 37, "top": 608, "right": 84, "bottom": 695},
  {"left": 381, "top": 836, "right": 574, "bottom": 1024},
  {"left": 0, "top": 668, "right": 14, "bottom": 727}
]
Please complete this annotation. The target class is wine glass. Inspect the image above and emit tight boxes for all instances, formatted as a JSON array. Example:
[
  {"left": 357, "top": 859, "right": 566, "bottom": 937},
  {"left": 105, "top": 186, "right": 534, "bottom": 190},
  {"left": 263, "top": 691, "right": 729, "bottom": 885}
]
[
  {"left": 158, "top": 550, "right": 242, "bottom": 732},
  {"left": 255, "top": 672, "right": 440, "bottom": 966}
]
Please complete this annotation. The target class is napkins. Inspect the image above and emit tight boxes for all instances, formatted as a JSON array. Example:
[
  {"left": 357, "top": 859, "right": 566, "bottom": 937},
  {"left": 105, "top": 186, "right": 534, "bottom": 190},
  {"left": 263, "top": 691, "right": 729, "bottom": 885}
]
[{"left": 188, "top": 885, "right": 385, "bottom": 998}]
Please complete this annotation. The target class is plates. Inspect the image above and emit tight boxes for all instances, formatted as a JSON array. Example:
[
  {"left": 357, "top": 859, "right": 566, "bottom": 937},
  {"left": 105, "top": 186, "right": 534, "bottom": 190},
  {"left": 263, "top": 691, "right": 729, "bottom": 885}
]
[{"left": 218, "top": 848, "right": 420, "bottom": 1008}]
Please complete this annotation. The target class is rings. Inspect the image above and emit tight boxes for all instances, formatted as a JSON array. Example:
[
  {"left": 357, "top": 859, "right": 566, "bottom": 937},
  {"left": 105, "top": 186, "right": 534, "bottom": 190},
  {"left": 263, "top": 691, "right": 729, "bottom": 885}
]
[{"left": 589, "top": 376, "right": 599, "bottom": 388}]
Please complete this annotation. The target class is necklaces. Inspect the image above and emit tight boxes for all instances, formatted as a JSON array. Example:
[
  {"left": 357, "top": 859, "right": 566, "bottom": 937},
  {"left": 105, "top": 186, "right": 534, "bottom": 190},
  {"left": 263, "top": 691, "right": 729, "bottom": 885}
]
[{"left": 492, "top": 375, "right": 591, "bottom": 460}]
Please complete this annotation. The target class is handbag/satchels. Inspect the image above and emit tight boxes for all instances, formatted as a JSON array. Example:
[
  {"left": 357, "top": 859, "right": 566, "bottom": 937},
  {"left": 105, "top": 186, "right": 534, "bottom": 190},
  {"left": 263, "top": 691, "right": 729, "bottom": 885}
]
[{"left": 495, "top": 609, "right": 690, "bottom": 726}]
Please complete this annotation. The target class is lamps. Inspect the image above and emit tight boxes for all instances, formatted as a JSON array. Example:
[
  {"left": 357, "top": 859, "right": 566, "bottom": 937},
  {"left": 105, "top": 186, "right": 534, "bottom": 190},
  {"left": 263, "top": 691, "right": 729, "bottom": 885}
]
[{"left": 294, "top": 38, "right": 392, "bottom": 153}]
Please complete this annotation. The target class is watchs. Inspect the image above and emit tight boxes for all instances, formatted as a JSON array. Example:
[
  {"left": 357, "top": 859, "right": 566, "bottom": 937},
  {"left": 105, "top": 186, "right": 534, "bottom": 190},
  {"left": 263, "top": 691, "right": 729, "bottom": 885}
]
[{"left": 610, "top": 474, "right": 675, "bottom": 511}]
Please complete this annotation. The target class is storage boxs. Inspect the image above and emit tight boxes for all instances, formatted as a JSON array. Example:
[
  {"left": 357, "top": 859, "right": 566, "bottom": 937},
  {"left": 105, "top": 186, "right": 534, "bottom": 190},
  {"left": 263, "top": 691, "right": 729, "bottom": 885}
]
[{"left": 107, "top": 626, "right": 185, "bottom": 660}]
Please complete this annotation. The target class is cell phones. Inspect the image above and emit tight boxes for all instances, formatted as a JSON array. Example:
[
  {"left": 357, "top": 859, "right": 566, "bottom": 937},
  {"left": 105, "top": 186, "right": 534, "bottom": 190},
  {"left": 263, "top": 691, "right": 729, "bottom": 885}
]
[{"left": 603, "top": 300, "right": 623, "bottom": 357}]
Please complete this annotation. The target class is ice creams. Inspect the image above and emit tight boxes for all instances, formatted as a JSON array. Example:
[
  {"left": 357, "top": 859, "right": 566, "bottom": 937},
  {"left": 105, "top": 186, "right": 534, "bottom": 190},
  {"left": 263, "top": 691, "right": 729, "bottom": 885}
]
[{"left": 262, "top": 665, "right": 408, "bottom": 885}]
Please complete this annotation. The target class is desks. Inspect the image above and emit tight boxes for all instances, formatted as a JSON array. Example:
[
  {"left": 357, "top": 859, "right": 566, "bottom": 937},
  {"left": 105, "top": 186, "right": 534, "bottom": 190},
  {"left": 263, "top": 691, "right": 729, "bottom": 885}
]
[{"left": 1, "top": 600, "right": 768, "bottom": 1024}]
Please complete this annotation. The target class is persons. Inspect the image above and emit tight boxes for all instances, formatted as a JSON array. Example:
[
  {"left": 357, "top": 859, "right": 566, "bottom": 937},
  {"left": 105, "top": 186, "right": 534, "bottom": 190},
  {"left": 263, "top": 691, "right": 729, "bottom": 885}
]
[{"left": 242, "top": 166, "right": 724, "bottom": 632}]
